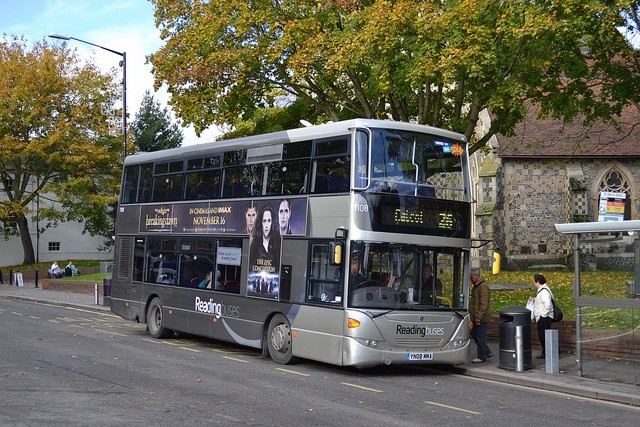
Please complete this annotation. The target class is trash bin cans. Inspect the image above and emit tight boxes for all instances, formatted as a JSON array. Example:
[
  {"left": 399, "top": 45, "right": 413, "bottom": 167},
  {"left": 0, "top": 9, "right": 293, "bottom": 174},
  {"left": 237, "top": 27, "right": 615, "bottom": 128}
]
[
  {"left": 498, "top": 306, "right": 533, "bottom": 370},
  {"left": 103, "top": 276, "right": 113, "bottom": 307}
]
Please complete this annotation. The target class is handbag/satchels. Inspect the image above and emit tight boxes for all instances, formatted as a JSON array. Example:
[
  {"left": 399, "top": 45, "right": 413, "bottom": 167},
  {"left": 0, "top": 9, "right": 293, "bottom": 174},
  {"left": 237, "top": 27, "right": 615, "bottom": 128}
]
[{"left": 536, "top": 289, "right": 563, "bottom": 322}]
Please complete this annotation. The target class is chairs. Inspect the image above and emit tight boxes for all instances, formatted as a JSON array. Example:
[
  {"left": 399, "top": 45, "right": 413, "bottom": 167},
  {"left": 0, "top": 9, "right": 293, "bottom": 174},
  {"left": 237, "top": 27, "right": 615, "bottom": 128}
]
[
  {"left": 250, "top": 179, "right": 262, "bottom": 196},
  {"left": 231, "top": 178, "right": 242, "bottom": 197},
  {"left": 326, "top": 169, "right": 350, "bottom": 193},
  {"left": 212, "top": 180, "right": 223, "bottom": 200},
  {"left": 165, "top": 184, "right": 181, "bottom": 200},
  {"left": 196, "top": 182, "right": 209, "bottom": 198},
  {"left": 129, "top": 188, "right": 149, "bottom": 203},
  {"left": 298, "top": 172, "right": 325, "bottom": 192},
  {"left": 419, "top": 180, "right": 435, "bottom": 197},
  {"left": 156, "top": 260, "right": 177, "bottom": 283},
  {"left": 223, "top": 280, "right": 239, "bottom": 291},
  {"left": 190, "top": 277, "right": 203, "bottom": 288}
]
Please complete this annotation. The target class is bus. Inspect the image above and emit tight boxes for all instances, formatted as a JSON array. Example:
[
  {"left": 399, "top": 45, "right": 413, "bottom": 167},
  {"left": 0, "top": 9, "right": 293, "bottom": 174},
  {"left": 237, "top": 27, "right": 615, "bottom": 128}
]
[{"left": 111, "top": 118, "right": 500, "bottom": 369}]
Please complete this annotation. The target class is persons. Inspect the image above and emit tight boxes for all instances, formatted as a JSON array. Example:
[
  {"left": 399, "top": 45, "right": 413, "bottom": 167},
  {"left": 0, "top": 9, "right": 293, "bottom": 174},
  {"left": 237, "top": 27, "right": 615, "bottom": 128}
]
[
  {"left": 529, "top": 274, "right": 562, "bottom": 359},
  {"left": 243, "top": 201, "right": 258, "bottom": 234},
  {"left": 337, "top": 250, "right": 370, "bottom": 296},
  {"left": 249, "top": 203, "right": 280, "bottom": 274},
  {"left": 469, "top": 267, "right": 494, "bottom": 363},
  {"left": 252, "top": 278, "right": 277, "bottom": 295},
  {"left": 65, "top": 260, "right": 75, "bottom": 277},
  {"left": 51, "top": 261, "right": 63, "bottom": 279},
  {"left": 198, "top": 271, "right": 212, "bottom": 289},
  {"left": 277, "top": 199, "right": 293, "bottom": 234},
  {"left": 415, "top": 264, "right": 442, "bottom": 303}
]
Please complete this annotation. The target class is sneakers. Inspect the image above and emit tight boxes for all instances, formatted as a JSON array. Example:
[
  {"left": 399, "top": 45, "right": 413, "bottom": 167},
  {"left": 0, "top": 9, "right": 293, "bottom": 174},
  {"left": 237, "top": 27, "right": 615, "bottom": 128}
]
[
  {"left": 472, "top": 359, "right": 485, "bottom": 363},
  {"left": 485, "top": 353, "right": 492, "bottom": 358}
]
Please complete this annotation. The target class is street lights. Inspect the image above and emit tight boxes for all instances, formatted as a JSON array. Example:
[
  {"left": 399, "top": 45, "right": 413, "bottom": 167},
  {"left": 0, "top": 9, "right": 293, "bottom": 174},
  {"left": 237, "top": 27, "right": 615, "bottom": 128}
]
[{"left": 48, "top": 33, "right": 132, "bottom": 164}]
